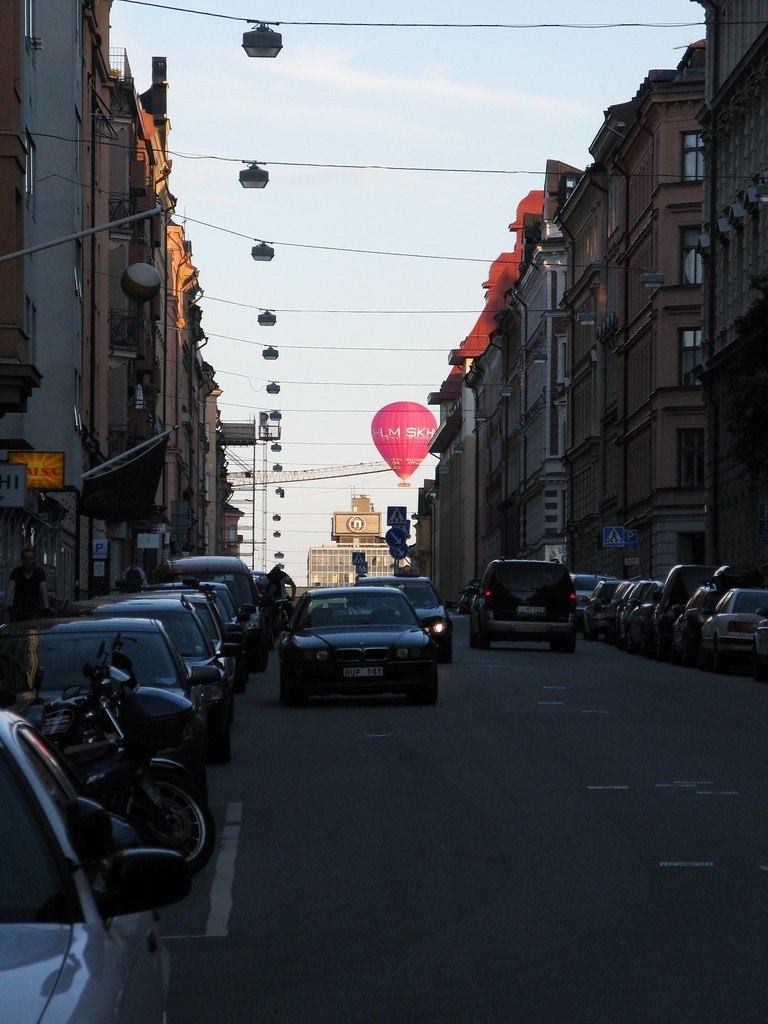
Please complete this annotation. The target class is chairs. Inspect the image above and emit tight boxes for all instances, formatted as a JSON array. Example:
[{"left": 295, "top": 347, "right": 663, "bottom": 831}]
[
  {"left": 306, "top": 608, "right": 334, "bottom": 627},
  {"left": 370, "top": 609, "right": 396, "bottom": 624}
]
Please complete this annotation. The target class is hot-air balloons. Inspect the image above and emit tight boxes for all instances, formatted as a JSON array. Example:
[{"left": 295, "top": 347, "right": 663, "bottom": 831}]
[{"left": 371, "top": 401, "right": 438, "bottom": 487}]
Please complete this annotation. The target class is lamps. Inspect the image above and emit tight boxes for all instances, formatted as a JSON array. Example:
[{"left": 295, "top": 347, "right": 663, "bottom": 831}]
[
  {"left": 269, "top": 407, "right": 284, "bottom": 569},
  {"left": 262, "top": 345, "right": 279, "bottom": 360},
  {"left": 241, "top": 19, "right": 284, "bottom": 57},
  {"left": 267, "top": 380, "right": 281, "bottom": 394},
  {"left": 258, "top": 308, "right": 277, "bottom": 326},
  {"left": 251, "top": 239, "right": 274, "bottom": 261},
  {"left": 238, "top": 160, "right": 269, "bottom": 188}
]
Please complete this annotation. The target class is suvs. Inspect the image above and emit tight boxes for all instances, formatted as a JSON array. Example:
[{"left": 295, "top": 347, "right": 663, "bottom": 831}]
[{"left": 463, "top": 555, "right": 578, "bottom": 653}]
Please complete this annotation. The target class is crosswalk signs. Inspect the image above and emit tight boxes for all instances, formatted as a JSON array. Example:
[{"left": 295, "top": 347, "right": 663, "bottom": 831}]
[
  {"left": 602, "top": 526, "right": 626, "bottom": 549},
  {"left": 351, "top": 551, "right": 366, "bottom": 564},
  {"left": 386, "top": 505, "right": 407, "bottom": 526},
  {"left": 356, "top": 561, "right": 368, "bottom": 574}
]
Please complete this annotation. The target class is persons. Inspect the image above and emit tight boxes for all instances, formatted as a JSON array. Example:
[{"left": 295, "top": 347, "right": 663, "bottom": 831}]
[
  {"left": 259, "top": 568, "right": 296, "bottom": 619},
  {"left": 4, "top": 549, "right": 49, "bottom": 623},
  {"left": 122, "top": 558, "right": 147, "bottom": 594}
]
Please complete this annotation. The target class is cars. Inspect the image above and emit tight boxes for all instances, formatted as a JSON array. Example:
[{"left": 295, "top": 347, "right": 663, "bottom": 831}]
[
  {"left": 3, "top": 613, "right": 221, "bottom": 814},
  {"left": 571, "top": 562, "right": 768, "bottom": 682},
  {"left": 278, "top": 586, "right": 441, "bottom": 706},
  {"left": 0, "top": 708, "right": 194, "bottom": 1024},
  {"left": 59, "top": 553, "right": 295, "bottom": 767},
  {"left": 346, "top": 576, "right": 459, "bottom": 665}
]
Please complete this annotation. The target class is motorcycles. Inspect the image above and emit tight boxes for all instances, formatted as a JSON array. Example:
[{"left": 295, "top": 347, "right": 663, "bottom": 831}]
[{"left": 5, "top": 638, "right": 216, "bottom": 877}]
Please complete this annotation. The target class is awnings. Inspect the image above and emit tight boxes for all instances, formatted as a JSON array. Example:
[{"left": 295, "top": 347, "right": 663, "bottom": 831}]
[{"left": 79, "top": 438, "right": 169, "bottom": 522}]
